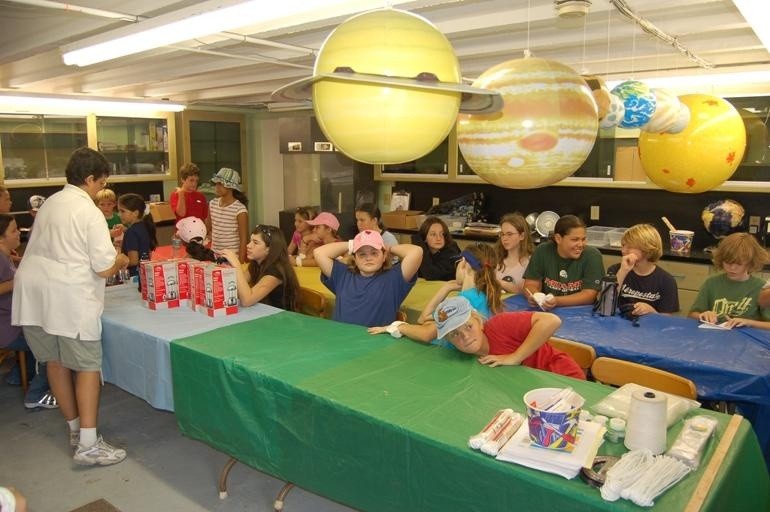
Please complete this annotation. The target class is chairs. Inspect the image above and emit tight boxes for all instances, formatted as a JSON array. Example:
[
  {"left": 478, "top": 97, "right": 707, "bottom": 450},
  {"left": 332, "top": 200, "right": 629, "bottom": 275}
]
[
  {"left": 1, "top": 350, "right": 28, "bottom": 395},
  {"left": 290, "top": 287, "right": 327, "bottom": 318},
  {"left": 396, "top": 310, "right": 408, "bottom": 323},
  {"left": 548, "top": 337, "right": 596, "bottom": 381},
  {"left": 589, "top": 355, "right": 696, "bottom": 433}
]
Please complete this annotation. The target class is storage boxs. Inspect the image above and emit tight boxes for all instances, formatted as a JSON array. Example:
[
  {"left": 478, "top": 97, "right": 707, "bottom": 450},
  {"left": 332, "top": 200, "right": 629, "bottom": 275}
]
[{"left": 599, "top": 139, "right": 650, "bottom": 181}]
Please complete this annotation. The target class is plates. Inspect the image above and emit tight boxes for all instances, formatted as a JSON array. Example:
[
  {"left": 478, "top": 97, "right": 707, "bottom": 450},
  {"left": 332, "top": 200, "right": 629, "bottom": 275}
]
[{"left": 535, "top": 210, "right": 560, "bottom": 237}]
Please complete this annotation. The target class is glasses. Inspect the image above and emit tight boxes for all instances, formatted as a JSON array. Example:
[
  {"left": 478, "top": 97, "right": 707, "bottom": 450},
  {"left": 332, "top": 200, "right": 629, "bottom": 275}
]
[{"left": 499, "top": 232, "right": 520, "bottom": 237}]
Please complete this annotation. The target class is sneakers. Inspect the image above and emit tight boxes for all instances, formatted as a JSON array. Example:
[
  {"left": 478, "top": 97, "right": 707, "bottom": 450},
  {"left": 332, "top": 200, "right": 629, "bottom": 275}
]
[
  {"left": 73, "top": 434, "right": 127, "bottom": 466},
  {"left": 68, "top": 430, "right": 79, "bottom": 447},
  {"left": 25, "top": 392, "right": 59, "bottom": 409}
]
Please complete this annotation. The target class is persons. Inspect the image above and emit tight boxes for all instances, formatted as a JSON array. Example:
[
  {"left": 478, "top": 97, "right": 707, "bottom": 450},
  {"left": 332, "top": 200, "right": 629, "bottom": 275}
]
[
  {"left": 607, "top": 223, "right": 681, "bottom": 317},
  {"left": 367, "top": 243, "right": 505, "bottom": 352},
  {"left": 0, "top": 216, "right": 60, "bottom": 410},
  {"left": 26, "top": 194, "right": 47, "bottom": 230},
  {"left": 170, "top": 163, "right": 210, "bottom": 238},
  {"left": 522, "top": 215, "right": 605, "bottom": 311},
  {"left": 433, "top": 295, "right": 587, "bottom": 384},
  {"left": 687, "top": 233, "right": 770, "bottom": 330},
  {"left": 11, "top": 148, "right": 130, "bottom": 466},
  {"left": 0, "top": 486, "right": 28, "bottom": 512},
  {"left": 417, "top": 217, "right": 464, "bottom": 280},
  {"left": 89, "top": 188, "right": 128, "bottom": 244},
  {"left": 289, "top": 211, "right": 344, "bottom": 267},
  {"left": 313, "top": 230, "right": 423, "bottom": 329},
  {"left": 207, "top": 167, "right": 248, "bottom": 264},
  {"left": 355, "top": 203, "right": 399, "bottom": 266},
  {"left": 490, "top": 213, "right": 534, "bottom": 294},
  {"left": 287, "top": 206, "right": 318, "bottom": 257},
  {"left": 0, "top": 184, "right": 13, "bottom": 214},
  {"left": 220, "top": 225, "right": 300, "bottom": 312},
  {"left": 176, "top": 217, "right": 228, "bottom": 267},
  {"left": 116, "top": 193, "right": 157, "bottom": 276}
]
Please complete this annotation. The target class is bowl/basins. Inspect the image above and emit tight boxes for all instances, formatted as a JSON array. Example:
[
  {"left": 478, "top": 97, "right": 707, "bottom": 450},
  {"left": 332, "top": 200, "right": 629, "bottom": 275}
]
[
  {"left": 525, "top": 212, "right": 539, "bottom": 230},
  {"left": 523, "top": 387, "right": 584, "bottom": 450},
  {"left": 668, "top": 229, "right": 695, "bottom": 257}
]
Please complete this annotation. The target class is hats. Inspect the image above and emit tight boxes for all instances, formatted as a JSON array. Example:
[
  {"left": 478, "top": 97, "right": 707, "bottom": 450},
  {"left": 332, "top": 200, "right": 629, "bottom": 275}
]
[
  {"left": 449, "top": 250, "right": 482, "bottom": 271},
  {"left": 304, "top": 212, "right": 340, "bottom": 231},
  {"left": 27, "top": 195, "right": 45, "bottom": 209},
  {"left": 209, "top": 168, "right": 241, "bottom": 192},
  {"left": 432, "top": 296, "right": 473, "bottom": 340},
  {"left": 177, "top": 216, "right": 207, "bottom": 243},
  {"left": 352, "top": 230, "right": 386, "bottom": 253}
]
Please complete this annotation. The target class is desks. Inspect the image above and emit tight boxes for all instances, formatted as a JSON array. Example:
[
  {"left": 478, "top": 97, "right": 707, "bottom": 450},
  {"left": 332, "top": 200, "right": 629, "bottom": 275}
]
[
  {"left": 100, "top": 284, "right": 286, "bottom": 412},
  {"left": 501, "top": 293, "right": 770, "bottom": 469},
  {"left": 170, "top": 308, "right": 769, "bottom": 512},
  {"left": 239, "top": 255, "right": 517, "bottom": 346}
]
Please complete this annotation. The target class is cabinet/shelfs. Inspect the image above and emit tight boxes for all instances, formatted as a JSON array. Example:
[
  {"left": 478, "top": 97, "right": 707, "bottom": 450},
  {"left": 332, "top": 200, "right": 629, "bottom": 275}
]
[
  {"left": 0, "top": 114, "right": 178, "bottom": 258},
  {"left": 279, "top": 116, "right": 379, "bottom": 242},
  {"left": 373, "top": 97, "right": 769, "bottom": 194}
]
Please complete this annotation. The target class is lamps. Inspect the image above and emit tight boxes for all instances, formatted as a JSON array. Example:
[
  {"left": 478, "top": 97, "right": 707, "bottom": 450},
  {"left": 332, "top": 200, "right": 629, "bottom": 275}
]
[
  {"left": 0, "top": 90, "right": 188, "bottom": 137},
  {"left": 59, "top": 0, "right": 405, "bottom": 68}
]
